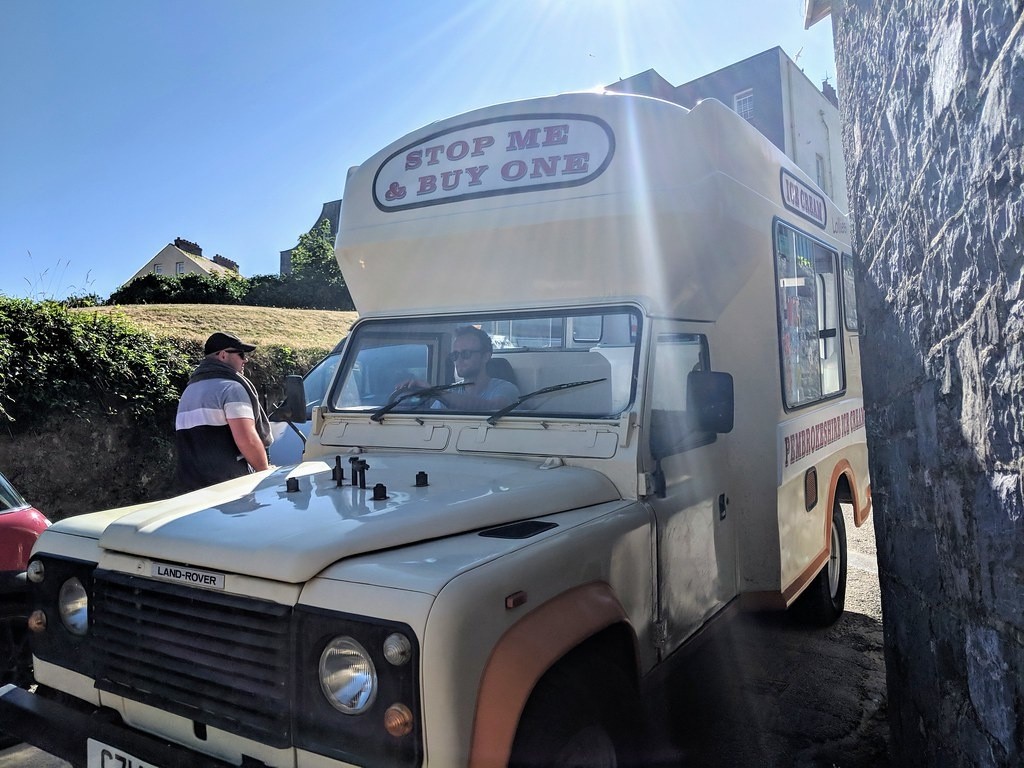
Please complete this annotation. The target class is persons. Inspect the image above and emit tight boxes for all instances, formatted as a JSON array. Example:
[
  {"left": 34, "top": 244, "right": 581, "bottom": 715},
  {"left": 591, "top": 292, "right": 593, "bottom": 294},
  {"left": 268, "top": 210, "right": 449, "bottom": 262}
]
[
  {"left": 393, "top": 326, "right": 521, "bottom": 410},
  {"left": 174, "top": 332, "right": 274, "bottom": 491}
]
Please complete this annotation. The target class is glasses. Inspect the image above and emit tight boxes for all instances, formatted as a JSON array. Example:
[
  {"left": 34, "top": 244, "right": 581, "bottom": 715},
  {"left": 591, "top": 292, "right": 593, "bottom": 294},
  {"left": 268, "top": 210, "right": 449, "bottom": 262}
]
[
  {"left": 216, "top": 350, "right": 244, "bottom": 360},
  {"left": 448, "top": 350, "right": 481, "bottom": 361}
]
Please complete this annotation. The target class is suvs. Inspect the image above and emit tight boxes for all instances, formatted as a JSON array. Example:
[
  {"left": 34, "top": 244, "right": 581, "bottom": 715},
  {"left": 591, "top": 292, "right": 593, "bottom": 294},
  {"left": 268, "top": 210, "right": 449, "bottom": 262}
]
[{"left": 252, "top": 333, "right": 513, "bottom": 473}]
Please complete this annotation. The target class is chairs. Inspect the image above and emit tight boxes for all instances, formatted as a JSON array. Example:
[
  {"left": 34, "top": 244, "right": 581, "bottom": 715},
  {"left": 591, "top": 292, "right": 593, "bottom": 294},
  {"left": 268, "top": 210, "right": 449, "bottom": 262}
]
[{"left": 485, "top": 358, "right": 518, "bottom": 386}]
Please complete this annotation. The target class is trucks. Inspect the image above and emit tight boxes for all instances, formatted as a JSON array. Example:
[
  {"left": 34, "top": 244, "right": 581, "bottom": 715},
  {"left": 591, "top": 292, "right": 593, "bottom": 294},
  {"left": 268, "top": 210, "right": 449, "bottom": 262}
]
[{"left": 0, "top": 42, "right": 869, "bottom": 768}]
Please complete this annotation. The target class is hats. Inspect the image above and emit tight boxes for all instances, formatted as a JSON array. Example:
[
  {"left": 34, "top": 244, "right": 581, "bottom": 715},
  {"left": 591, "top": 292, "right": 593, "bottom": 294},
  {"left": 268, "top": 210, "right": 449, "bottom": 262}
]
[{"left": 204, "top": 333, "right": 256, "bottom": 352}]
